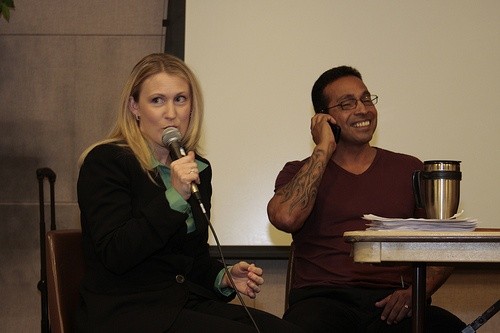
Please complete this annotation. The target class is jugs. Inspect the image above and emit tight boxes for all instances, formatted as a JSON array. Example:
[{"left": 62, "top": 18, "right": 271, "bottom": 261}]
[{"left": 412, "top": 160, "right": 462, "bottom": 219}]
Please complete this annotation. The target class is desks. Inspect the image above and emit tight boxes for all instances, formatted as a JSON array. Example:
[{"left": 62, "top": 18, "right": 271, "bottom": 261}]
[{"left": 342, "top": 230, "right": 500, "bottom": 333}]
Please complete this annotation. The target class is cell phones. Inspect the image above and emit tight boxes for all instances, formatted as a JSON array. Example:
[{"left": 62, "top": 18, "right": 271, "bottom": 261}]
[{"left": 327, "top": 120, "right": 341, "bottom": 144}]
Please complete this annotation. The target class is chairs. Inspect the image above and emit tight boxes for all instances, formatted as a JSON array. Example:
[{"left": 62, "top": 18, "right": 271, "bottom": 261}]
[{"left": 45, "top": 229, "right": 83, "bottom": 333}]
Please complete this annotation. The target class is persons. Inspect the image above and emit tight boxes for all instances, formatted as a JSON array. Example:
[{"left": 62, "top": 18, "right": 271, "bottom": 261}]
[
  {"left": 266, "top": 65, "right": 468, "bottom": 333},
  {"left": 76, "top": 53, "right": 282, "bottom": 333}
]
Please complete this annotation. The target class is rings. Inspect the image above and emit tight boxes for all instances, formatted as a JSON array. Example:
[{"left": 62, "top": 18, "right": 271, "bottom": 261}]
[
  {"left": 403, "top": 304, "right": 410, "bottom": 308},
  {"left": 190, "top": 167, "right": 196, "bottom": 174}
]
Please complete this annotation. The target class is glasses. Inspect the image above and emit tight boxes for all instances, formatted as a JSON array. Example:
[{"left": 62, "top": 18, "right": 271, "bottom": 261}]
[{"left": 321, "top": 95, "right": 378, "bottom": 112}]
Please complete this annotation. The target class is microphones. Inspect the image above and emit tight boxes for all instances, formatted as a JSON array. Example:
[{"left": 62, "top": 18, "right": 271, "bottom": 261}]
[{"left": 162, "top": 126, "right": 203, "bottom": 204}]
